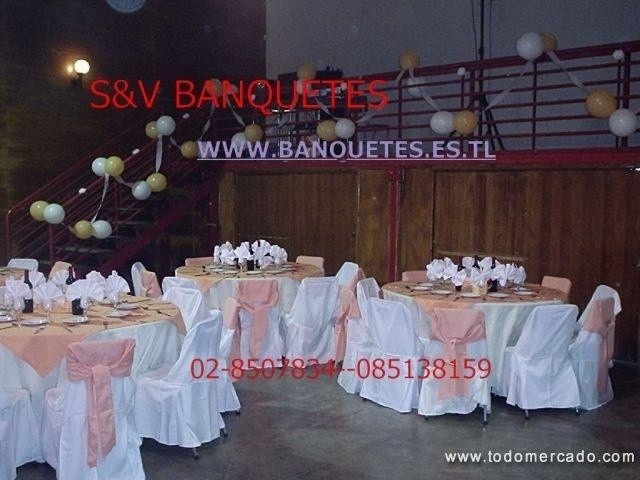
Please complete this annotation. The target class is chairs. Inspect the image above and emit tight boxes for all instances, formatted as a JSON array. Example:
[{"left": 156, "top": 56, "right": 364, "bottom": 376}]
[
  {"left": 337, "top": 277, "right": 381, "bottom": 395},
  {"left": 0, "top": 389, "right": 43, "bottom": 480},
  {"left": 506, "top": 303, "right": 583, "bottom": 420},
  {"left": 217, "top": 296, "right": 241, "bottom": 417},
  {"left": 571, "top": 298, "right": 616, "bottom": 411},
  {"left": 286, "top": 278, "right": 336, "bottom": 368},
  {"left": 239, "top": 280, "right": 283, "bottom": 370},
  {"left": 162, "top": 287, "right": 202, "bottom": 332},
  {"left": 46, "top": 339, "right": 146, "bottom": 480},
  {"left": 578, "top": 283, "right": 621, "bottom": 328},
  {"left": 358, "top": 297, "right": 418, "bottom": 413},
  {"left": 418, "top": 308, "right": 490, "bottom": 426},
  {"left": 137, "top": 310, "right": 229, "bottom": 459}
]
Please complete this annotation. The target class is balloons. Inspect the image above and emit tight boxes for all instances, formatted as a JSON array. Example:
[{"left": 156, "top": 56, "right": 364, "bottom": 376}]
[
  {"left": 609, "top": 110, "right": 637, "bottom": 136},
  {"left": 132, "top": 181, "right": 152, "bottom": 201},
  {"left": 516, "top": 32, "right": 543, "bottom": 61},
  {"left": 316, "top": 121, "right": 338, "bottom": 141},
  {"left": 93, "top": 221, "right": 112, "bottom": 238},
  {"left": 408, "top": 77, "right": 425, "bottom": 98},
  {"left": 542, "top": 33, "right": 557, "bottom": 50},
  {"left": 431, "top": 110, "right": 456, "bottom": 135},
  {"left": 455, "top": 111, "right": 475, "bottom": 134},
  {"left": 297, "top": 62, "right": 317, "bottom": 80},
  {"left": 105, "top": 156, "right": 125, "bottom": 176},
  {"left": 74, "top": 220, "right": 94, "bottom": 240},
  {"left": 43, "top": 204, "right": 65, "bottom": 224},
  {"left": 232, "top": 132, "right": 249, "bottom": 150},
  {"left": 587, "top": 90, "right": 617, "bottom": 120},
  {"left": 147, "top": 174, "right": 166, "bottom": 192},
  {"left": 399, "top": 51, "right": 419, "bottom": 69},
  {"left": 206, "top": 79, "right": 224, "bottom": 96},
  {"left": 182, "top": 141, "right": 199, "bottom": 158},
  {"left": 30, "top": 201, "right": 49, "bottom": 222},
  {"left": 297, "top": 79, "right": 311, "bottom": 95},
  {"left": 146, "top": 121, "right": 161, "bottom": 139},
  {"left": 226, "top": 84, "right": 240, "bottom": 95},
  {"left": 92, "top": 158, "right": 106, "bottom": 177},
  {"left": 335, "top": 118, "right": 355, "bottom": 138},
  {"left": 245, "top": 124, "right": 263, "bottom": 142},
  {"left": 158, "top": 117, "right": 175, "bottom": 135}
]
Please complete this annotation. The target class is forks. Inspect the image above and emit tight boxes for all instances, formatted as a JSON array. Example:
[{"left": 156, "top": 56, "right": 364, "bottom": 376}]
[
  {"left": 103, "top": 320, "right": 108, "bottom": 330},
  {"left": 156, "top": 309, "right": 170, "bottom": 317},
  {"left": 34, "top": 325, "right": 46, "bottom": 334}
]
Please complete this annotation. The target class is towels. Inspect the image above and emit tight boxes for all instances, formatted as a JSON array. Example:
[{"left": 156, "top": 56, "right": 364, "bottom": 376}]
[
  {"left": 514, "top": 267, "right": 527, "bottom": 285},
  {"left": 86, "top": 272, "right": 107, "bottom": 299},
  {"left": 35, "top": 283, "right": 61, "bottom": 305},
  {"left": 444, "top": 258, "right": 458, "bottom": 281},
  {"left": 480, "top": 257, "right": 491, "bottom": 269},
  {"left": 507, "top": 263, "right": 519, "bottom": 281},
  {"left": 426, "top": 260, "right": 443, "bottom": 279},
  {"left": 270, "top": 246, "right": 288, "bottom": 263},
  {"left": 236, "top": 243, "right": 253, "bottom": 263},
  {"left": 105, "top": 275, "right": 129, "bottom": 303},
  {"left": 456, "top": 270, "right": 467, "bottom": 286},
  {"left": 220, "top": 242, "right": 233, "bottom": 251},
  {"left": 6, "top": 281, "right": 31, "bottom": 309},
  {"left": 67, "top": 280, "right": 89, "bottom": 302},
  {"left": 252, "top": 240, "right": 270, "bottom": 256},
  {"left": 51, "top": 271, "right": 68, "bottom": 287},
  {"left": 221, "top": 251, "right": 236, "bottom": 263},
  {"left": 461, "top": 256, "right": 474, "bottom": 269},
  {"left": 212, "top": 246, "right": 220, "bottom": 262},
  {"left": 492, "top": 265, "right": 507, "bottom": 285},
  {"left": 472, "top": 270, "right": 488, "bottom": 288}
]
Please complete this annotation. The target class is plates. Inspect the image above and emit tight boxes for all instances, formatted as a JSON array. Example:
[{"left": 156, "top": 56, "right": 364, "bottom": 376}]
[
  {"left": 0, "top": 310, "right": 16, "bottom": 322},
  {"left": 246, "top": 270, "right": 263, "bottom": 275},
  {"left": 430, "top": 289, "right": 452, "bottom": 295},
  {"left": 107, "top": 310, "right": 131, "bottom": 318},
  {"left": 206, "top": 269, "right": 223, "bottom": 273},
  {"left": 510, "top": 285, "right": 529, "bottom": 292},
  {"left": 118, "top": 303, "right": 139, "bottom": 309},
  {"left": 488, "top": 291, "right": 510, "bottom": 299},
  {"left": 264, "top": 269, "right": 279, "bottom": 275},
  {"left": 224, "top": 270, "right": 238, "bottom": 274},
  {"left": 20, "top": 318, "right": 45, "bottom": 325},
  {"left": 280, "top": 263, "right": 295, "bottom": 272},
  {"left": 516, "top": 291, "right": 537, "bottom": 297},
  {"left": 459, "top": 293, "right": 481, "bottom": 299},
  {"left": 63, "top": 317, "right": 89, "bottom": 324},
  {"left": 411, "top": 282, "right": 434, "bottom": 292}
]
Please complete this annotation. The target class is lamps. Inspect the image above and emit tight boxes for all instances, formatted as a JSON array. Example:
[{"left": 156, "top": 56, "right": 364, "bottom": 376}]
[{"left": 71, "top": 59, "right": 90, "bottom": 97}]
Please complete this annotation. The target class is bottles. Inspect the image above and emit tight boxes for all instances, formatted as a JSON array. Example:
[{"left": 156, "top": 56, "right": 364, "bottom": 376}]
[
  {"left": 455, "top": 256, "right": 466, "bottom": 292},
  {"left": 247, "top": 242, "right": 254, "bottom": 271},
  {"left": 487, "top": 257, "right": 497, "bottom": 292},
  {"left": 72, "top": 273, "right": 84, "bottom": 315},
  {"left": 472, "top": 255, "right": 480, "bottom": 270},
  {"left": 256, "top": 240, "right": 261, "bottom": 269},
  {"left": 22, "top": 269, "right": 33, "bottom": 314},
  {"left": 66, "top": 266, "right": 76, "bottom": 294},
  {"left": 236, "top": 257, "right": 241, "bottom": 269}
]
